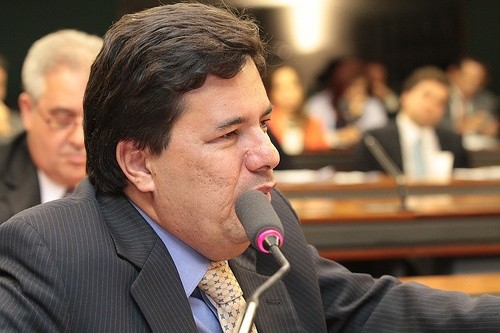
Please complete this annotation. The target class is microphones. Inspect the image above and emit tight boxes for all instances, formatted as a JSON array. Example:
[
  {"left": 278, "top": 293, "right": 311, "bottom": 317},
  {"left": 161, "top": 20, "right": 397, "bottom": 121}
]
[{"left": 233, "top": 189, "right": 290, "bottom": 333}]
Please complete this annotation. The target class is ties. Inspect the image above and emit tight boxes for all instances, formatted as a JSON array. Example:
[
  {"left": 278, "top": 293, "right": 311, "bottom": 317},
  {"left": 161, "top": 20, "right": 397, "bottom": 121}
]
[
  {"left": 409, "top": 139, "right": 425, "bottom": 177},
  {"left": 196, "top": 260, "right": 258, "bottom": 333}
]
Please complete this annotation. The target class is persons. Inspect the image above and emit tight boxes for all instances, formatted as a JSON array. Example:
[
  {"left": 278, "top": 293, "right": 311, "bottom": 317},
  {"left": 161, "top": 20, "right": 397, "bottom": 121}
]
[
  {"left": 365, "top": 61, "right": 401, "bottom": 115},
  {"left": 302, "top": 57, "right": 388, "bottom": 150},
  {"left": 0, "top": 4, "right": 500, "bottom": 332},
  {"left": 1, "top": 58, "right": 28, "bottom": 143},
  {"left": 361, "top": 66, "right": 469, "bottom": 278},
  {"left": 0, "top": 29, "right": 106, "bottom": 223},
  {"left": 448, "top": 58, "right": 499, "bottom": 163},
  {"left": 259, "top": 55, "right": 362, "bottom": 275}
]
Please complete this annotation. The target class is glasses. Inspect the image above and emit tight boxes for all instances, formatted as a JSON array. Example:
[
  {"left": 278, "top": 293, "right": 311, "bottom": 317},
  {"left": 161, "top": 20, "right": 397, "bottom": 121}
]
[{"left": 32, "top": 101, "right": 84, "bottom": 134}]
